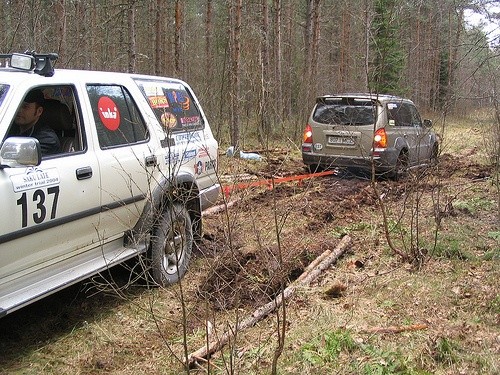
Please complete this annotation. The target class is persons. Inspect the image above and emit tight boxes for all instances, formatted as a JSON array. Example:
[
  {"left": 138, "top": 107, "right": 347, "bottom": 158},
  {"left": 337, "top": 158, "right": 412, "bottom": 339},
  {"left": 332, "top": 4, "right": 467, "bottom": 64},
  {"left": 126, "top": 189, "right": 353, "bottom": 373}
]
[{"left": 7, "top": 88, "right": 61, "bottom": 156}]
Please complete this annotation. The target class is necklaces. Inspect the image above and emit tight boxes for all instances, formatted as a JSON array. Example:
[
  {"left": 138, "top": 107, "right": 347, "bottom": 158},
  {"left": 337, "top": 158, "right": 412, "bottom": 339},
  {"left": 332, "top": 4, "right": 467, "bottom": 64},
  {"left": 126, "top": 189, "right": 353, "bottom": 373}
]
[{"left": 29, "top": 124, "right": 34, "bottom": 137}]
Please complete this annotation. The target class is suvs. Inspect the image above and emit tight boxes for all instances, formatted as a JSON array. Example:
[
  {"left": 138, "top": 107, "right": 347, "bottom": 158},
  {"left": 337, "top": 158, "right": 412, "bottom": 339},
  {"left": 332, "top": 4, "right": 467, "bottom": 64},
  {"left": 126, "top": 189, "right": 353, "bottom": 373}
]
[
  {"left": 0, "top": 50, "right": 220, "bottom": 322},
  {"left": 301, "top": 92, "right": 440, "bottom": 182}
]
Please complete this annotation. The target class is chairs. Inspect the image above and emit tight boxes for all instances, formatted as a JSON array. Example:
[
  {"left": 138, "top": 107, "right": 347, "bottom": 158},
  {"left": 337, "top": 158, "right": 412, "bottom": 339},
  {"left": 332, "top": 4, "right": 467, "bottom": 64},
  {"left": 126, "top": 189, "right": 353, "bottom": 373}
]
[{"left": 43, "top": 99, "right": 75, "bottom": 153}]
